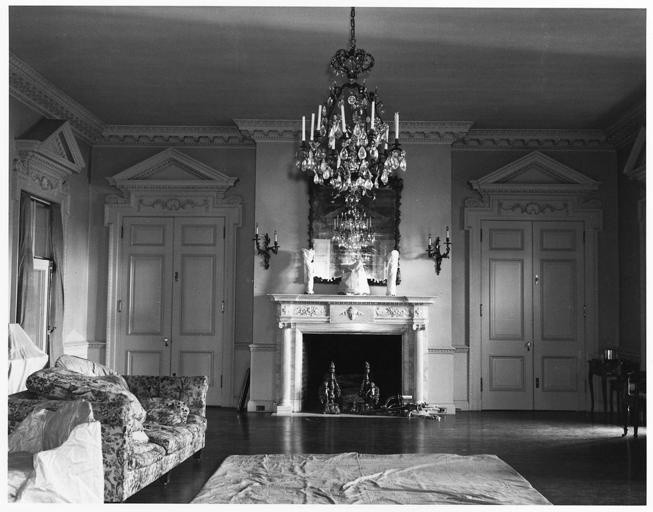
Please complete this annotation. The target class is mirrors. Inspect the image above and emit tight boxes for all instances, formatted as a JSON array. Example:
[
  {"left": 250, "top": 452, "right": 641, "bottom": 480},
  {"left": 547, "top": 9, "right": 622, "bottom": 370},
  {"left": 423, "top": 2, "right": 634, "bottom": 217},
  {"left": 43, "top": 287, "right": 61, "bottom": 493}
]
[{"left": 306, "top": 170, "right": 403, "bottom": 286}]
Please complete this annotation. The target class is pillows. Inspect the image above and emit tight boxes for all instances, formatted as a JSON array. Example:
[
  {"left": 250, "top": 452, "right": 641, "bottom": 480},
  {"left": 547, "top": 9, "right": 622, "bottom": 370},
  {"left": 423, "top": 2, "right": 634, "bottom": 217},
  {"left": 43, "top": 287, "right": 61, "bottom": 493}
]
[{"left": 142, "top": 396, "right": 189, "bottom": 429}]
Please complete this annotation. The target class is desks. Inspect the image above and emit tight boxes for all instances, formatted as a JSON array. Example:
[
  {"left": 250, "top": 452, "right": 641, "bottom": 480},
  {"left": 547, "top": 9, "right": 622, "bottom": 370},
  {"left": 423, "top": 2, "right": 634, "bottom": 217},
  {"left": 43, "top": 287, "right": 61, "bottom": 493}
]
[{"left": 585, "top": 356, "right": 640, "bottom": 421}]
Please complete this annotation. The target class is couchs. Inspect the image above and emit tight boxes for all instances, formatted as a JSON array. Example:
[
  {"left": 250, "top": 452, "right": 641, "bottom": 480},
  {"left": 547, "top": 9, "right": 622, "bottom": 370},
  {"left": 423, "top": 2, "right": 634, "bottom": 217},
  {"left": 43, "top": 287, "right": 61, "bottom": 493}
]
[{"left": 8, "top": 352, "right": 210, "bottom": 504}]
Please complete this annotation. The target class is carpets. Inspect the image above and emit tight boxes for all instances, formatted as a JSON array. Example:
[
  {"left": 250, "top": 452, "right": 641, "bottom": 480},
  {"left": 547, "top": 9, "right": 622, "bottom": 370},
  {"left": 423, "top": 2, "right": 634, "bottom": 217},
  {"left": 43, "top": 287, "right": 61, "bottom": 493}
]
[{"left": 188, "top": 450, "right": 553, "bottom": 504}]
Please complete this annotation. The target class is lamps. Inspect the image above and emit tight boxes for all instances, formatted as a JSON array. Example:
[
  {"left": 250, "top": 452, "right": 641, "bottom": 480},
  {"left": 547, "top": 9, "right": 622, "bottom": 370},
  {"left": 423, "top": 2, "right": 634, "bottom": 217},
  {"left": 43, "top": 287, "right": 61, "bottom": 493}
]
[
  {"left": 251, "top": 223, "right": 281, "bottom": 271},
  {"left": 293, "top": 7, "right": 409, "bottom": 208},
  {"left": 332, "top": 205, "right": 376, "bottom": 251},
  {"left": 425, "top": 226, "right": 453, "bottom": 277}
]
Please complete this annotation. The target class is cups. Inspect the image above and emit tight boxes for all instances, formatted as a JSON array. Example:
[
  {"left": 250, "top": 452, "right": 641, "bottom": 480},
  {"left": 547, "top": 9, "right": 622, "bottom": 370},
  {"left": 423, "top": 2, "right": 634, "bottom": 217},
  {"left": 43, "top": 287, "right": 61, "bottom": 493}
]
[{"left": 604, "top": 349, "right": 617, "bottom": 360}]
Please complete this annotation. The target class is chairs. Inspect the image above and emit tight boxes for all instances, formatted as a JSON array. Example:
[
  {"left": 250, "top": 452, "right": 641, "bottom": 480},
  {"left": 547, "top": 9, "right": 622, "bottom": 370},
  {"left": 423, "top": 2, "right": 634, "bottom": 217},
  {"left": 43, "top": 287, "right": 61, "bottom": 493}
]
[
  {"left": 8, "top": 398, "right": 107, "bottom": 504},
  {"left": 619, "top": 358, "right": 646, "bottom": 438}
]
